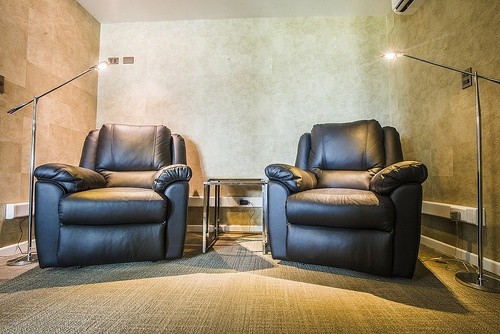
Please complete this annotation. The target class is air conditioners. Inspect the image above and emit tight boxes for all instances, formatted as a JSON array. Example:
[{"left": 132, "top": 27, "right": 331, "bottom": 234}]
[{"left": 390, "top": 0, "right": 426, "bottom": 16}]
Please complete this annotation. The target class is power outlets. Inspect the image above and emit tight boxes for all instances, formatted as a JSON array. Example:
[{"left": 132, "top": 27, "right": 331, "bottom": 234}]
[{"left": 237, "top": 198, "right": 250, "bottom": 205}]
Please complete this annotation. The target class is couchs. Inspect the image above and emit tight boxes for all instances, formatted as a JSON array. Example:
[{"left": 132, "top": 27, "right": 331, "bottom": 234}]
[
  {"left": 264, "top": 118, "right": 427, "bottom": 279},
  {"left": 34, "top": 123, "right": 193, "bottom": 269}
]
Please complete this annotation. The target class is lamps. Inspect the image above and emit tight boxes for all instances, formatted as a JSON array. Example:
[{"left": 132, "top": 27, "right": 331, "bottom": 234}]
[
  {"left": 6, "top": 60, "right": 110, "bottom": 267},
  {"left": 384, "top": 50, "right": 500, "bottom": 294}
]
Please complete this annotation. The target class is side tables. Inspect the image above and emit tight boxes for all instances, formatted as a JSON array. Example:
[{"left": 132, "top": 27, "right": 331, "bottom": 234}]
[{"left": 201, "top": 177, "right": 268, "bottom": 253}]
